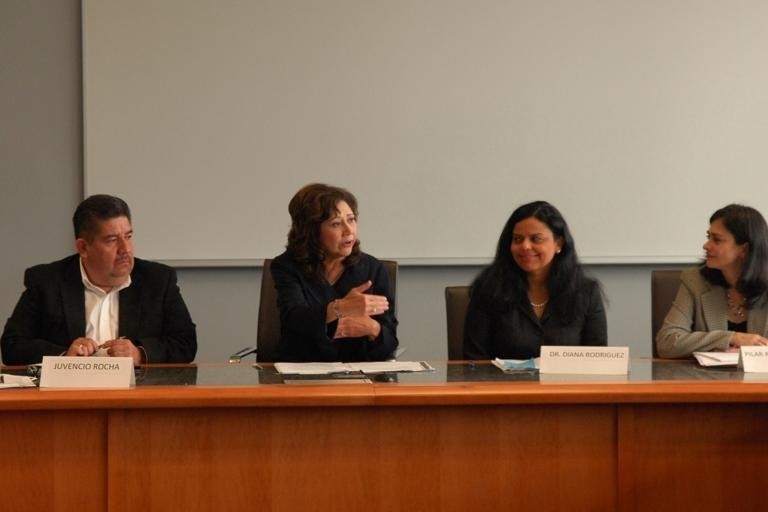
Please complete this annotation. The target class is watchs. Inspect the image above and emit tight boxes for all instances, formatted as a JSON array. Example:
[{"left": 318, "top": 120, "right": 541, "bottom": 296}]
[{"left": 333, "top": 301, "right": 343, "bottom": 319}]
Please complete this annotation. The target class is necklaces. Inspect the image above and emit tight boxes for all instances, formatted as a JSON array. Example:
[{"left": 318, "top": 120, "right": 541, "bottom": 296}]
[
  {"left": 725, "top": 291, "right": 751, "bottom": 318},
  {"left": 529, "top": 300, "right": 549, "bottom": 308}
]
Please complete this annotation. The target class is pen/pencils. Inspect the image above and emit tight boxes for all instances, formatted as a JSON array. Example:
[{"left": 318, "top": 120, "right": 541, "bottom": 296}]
[
  {"left": 468, "top": 362, "right": 478, "bottom": 368},
  {"left": 97, "top": 335, "right": 128, "bottom": 348},
  {"left": 253, "top": 362, "right": 264, "bottom": 371}
]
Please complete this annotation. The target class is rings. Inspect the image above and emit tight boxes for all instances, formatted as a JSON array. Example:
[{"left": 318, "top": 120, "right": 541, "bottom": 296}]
[{"left": 79, "top": 343, "right": 84, "bottom": 349}]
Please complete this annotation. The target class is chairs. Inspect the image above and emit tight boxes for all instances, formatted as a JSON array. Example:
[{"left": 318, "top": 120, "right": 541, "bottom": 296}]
[
  {"left": 444, "top": 285, "right": 473, "bottom": 361},
  {"left": 255, "top": 258, "right": 398, "bottom": 366},
  {"left": 648, "top": 268, "right": 682, "bottom": 360}
]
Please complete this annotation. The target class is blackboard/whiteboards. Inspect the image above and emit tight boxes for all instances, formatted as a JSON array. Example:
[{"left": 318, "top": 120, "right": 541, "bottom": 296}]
[{"left": 83, "top": 0, "right": 768, "bottom": 269}]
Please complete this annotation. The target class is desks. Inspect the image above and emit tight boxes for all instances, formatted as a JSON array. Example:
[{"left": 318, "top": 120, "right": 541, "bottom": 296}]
[{"left": 0, "top": 358, "right": 767, "bottom": 512}]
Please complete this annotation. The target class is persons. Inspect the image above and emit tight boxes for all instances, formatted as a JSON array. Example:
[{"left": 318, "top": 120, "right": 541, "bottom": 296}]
[
  {"left": 469, "top": 202, "right": 608, "bottom": 359},
  {"left": 654, "top": 204, "right": 767, "bottom": 356},
  {"left": 267, "top": 184, "right": 400, "bottom": 359},
  {"left": 2, "top": 194, "right": 199, "bottom": 366}
]
